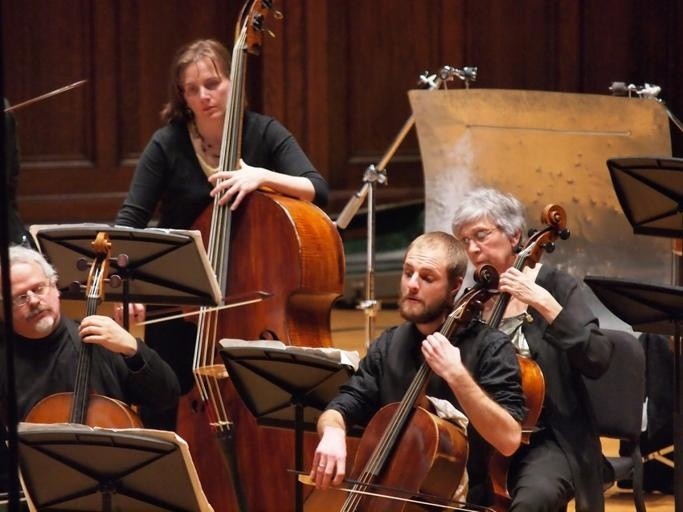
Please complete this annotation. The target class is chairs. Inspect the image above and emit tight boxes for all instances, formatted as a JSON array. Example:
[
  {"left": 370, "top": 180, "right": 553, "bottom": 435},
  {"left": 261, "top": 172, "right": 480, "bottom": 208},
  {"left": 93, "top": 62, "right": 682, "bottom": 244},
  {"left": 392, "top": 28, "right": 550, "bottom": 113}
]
[{"left": 583, "top": 328, "right": 646, "bottom": 512}]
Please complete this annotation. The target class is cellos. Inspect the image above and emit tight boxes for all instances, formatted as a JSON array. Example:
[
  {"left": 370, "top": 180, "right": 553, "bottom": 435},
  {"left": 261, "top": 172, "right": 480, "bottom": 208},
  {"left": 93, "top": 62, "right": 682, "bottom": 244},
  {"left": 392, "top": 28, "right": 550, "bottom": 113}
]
[
  {"left": 468, "top": 204, "right": 570, "bottom": 511},
  {"left": 303, "top": 262, "right": 499, "bottom": 511},
  {"left": 177, "top": 1, "right": 347, "bottom": 512},
  {"left": 24, "top": 232, "right": 148, "bottom": 429}
]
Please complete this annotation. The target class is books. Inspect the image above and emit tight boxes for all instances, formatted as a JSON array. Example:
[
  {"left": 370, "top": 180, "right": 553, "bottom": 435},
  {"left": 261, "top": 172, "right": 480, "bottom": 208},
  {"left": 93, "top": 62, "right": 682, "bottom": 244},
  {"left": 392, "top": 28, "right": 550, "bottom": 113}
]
[
  {"left": 14, "top": 419, "right": 215, "bottom": 512},
  {"left": 28, "top": 220, "right": 222, "bottom": 305},
  {"left": 219, "top": 334, "right": 361, "bottom": 371}
]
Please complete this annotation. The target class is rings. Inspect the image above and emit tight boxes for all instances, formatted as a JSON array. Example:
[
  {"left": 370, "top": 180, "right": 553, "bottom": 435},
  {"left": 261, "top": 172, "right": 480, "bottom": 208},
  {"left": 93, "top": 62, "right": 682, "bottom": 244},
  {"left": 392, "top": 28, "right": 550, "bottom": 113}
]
[{"left": 317, "top": 462, "right": 326, "bottom": 468}]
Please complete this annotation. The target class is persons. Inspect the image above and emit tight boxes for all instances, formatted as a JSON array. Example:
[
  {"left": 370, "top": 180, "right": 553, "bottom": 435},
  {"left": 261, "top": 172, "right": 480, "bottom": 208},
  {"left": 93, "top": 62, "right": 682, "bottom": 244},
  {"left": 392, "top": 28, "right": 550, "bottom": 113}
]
[
  {"left": 6, "top": 245, "right": 180, "bottom": 432},
  {"left": 450, "top": 186, "right": 613, "bottom": 511},
  {"left": 114, "top": 39, "right": 328, "bottom": 397},
  {"left": 308, "top": 231, "right": 528, "bottom": 511}
]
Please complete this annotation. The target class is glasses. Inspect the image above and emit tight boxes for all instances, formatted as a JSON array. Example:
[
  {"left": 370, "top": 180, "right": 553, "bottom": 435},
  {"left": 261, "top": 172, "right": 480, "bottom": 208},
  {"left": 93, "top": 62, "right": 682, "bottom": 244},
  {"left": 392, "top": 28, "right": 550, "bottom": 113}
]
[
  {"left": 10, "top": 281, "right": 53, "bottom": 307},
  {"left": 462, "top": 227, "right": 496, "bottom": 246}
]
